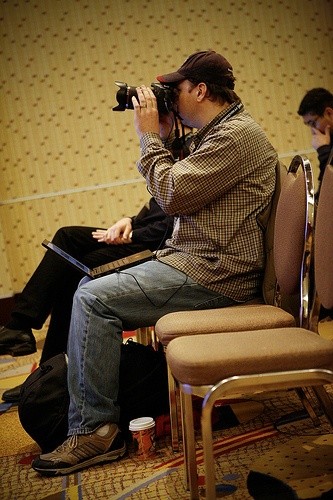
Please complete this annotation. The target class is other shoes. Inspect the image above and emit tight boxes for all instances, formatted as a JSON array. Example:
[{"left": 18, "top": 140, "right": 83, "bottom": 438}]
[
  {"left": 0, "top": 329, "right": 37, "bottom": 357},
  {"left": 1, "top": 384, "right": 22, "bottom": 402}
]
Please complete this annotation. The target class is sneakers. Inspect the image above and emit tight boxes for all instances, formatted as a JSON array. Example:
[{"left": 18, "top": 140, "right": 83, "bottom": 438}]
[{"left": 31, "top": 422, "right": 126, "bottom": 477}]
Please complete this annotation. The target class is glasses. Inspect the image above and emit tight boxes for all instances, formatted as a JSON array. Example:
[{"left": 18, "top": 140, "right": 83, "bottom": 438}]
[{"left": 304, "top": 113, "right": 323, "bottom": 128}]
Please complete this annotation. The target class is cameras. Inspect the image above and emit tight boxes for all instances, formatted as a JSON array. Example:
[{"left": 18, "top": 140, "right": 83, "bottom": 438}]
[{"left": 112, "top": 81, "right": 176, "bottom": 114}]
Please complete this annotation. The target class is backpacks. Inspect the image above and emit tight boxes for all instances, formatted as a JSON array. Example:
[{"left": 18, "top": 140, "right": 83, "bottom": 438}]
[{"left": 18, "top": 338, "right": 169, "bottom": 453}]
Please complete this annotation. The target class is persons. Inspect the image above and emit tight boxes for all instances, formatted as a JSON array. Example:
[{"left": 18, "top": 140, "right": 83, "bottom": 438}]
[
  {"left": 30, "top": 48, "right": 278, "bottom": 476},
  {"left": 297, "top": 87, "right": 333, "bottom": 202},
  {"left": 1, "top": 83, "right": 193, "bottom": 400}
]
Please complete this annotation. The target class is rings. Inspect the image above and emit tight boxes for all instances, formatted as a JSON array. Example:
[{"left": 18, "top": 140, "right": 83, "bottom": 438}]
[{"left": 140, "top": 106, "right": 147, "bottom": 108}]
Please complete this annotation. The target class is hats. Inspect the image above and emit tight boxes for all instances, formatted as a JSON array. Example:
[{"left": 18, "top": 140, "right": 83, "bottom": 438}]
[{"left": 156, "top": 51, "right": 235, "bottom": 91}]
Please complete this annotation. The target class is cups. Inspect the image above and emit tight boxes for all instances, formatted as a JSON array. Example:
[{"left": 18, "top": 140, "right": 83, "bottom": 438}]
[{"left": 129, "top": 417, "right": 156, "bottom": 462}]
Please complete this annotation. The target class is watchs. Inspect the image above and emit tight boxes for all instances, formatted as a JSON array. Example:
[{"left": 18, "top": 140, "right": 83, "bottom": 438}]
[{"left": 129, "top": 230, "right": 133, "bottom": 241}]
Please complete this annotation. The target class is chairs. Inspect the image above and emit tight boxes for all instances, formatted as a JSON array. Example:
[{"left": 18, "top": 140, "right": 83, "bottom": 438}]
[{"left": 141, "top": 153, "right": 333, "bottom": 500}]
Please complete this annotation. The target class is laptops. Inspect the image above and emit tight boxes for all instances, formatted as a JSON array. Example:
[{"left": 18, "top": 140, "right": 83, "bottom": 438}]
[{"left": 42, "top": 239, "right": 157, "bottom": 280}]
